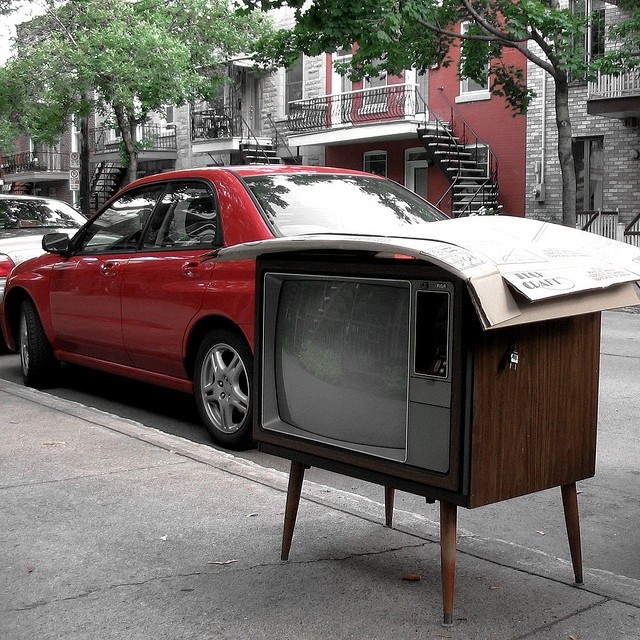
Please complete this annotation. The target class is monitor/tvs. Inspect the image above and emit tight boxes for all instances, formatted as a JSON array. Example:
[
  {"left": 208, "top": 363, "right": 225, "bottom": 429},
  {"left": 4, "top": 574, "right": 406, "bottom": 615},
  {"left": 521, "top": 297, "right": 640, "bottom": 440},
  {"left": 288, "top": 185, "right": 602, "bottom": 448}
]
[{"left": 251, "top": 249, "right": 602, "bottom": 510}]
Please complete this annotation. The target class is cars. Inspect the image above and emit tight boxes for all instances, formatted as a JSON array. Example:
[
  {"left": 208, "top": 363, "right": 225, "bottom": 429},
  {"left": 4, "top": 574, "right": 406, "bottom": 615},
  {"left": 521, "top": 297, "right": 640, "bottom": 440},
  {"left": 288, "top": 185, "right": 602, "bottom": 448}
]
[
  {"left": 1, "top": 194, "right": 124, "bottom": 301},
  {"left": 0, "top": 165, "right": 452, "bottom": 451}
]
